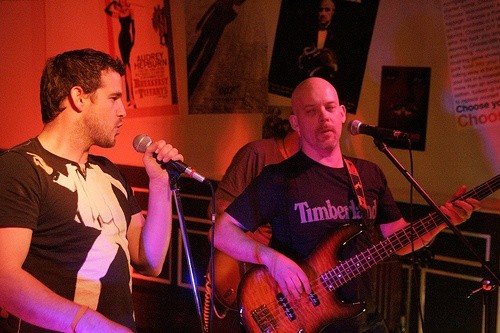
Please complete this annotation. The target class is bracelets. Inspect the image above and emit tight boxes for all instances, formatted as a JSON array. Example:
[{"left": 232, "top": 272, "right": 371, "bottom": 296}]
[{"left": 71, "top": 305, "right": 89, "bottom": 333}]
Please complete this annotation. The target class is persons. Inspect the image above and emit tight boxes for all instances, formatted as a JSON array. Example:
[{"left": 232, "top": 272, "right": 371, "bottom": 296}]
[
  {"left": 0, "top": 48, "right": 184, "bottom": 333},
  {"left": 208, "top": 126, "right": 303, "bottom": 332},
  {"left": 210, "top": 78, "right": 480, "bottom": 333},
  {"left": 295, "top": 2, "right": 337, "bottom": 77}
]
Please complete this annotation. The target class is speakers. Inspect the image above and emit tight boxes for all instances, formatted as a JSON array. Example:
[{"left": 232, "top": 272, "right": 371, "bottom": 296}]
[
  {"left": 132, "top": 228, "right": 177, "bottom": 283},
  {"left": 131, "top": 186, "right": 215, "bottom": 226},
  {"left": 428, "top": 228, "right": 499, "bottom": 268},
  {"left": 178, "top": 228, "right": 211, "bottom": 291},
  {"left": 417, "top": 264, "right": 498, "bottom": 333}
]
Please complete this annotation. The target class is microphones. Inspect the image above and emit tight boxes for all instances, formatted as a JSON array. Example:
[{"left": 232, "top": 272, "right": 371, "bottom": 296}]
[
  {"left": 348, "top": 119, "right": 406, "bottom": 139},
  {"left": 133, "top": 135, "right": 206, "bottom": 184}
]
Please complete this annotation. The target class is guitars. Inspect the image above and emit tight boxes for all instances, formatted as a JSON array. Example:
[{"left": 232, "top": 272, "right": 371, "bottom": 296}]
[
  {"left": 236, "top": 173, "right": 500, "bottom": 333},
  {"left": 206, "top": 220, "right": 271, "bottom": 311}
]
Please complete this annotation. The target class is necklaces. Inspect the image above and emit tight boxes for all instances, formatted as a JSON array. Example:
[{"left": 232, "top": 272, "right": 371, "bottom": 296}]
[{"left": 282, "top": 137, "right": 289, "bottom": 159}]
